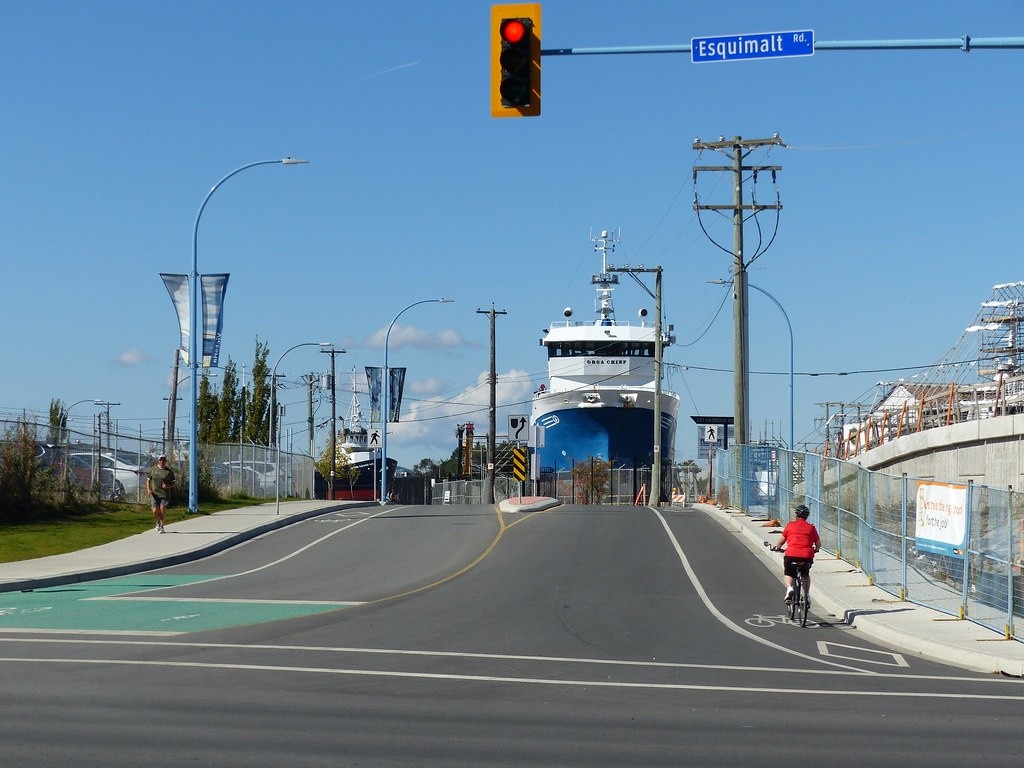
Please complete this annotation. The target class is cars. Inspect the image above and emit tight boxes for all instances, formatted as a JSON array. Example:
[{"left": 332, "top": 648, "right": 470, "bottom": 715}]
[
  {"left": 174, "top": 461, "right": 259, "bottom": 498},
  {"left": 0, "top": 439, "right": 92, "bottom": 487},
  {"left": 70, "top": 452, "right": 148, "bottom": 502}
]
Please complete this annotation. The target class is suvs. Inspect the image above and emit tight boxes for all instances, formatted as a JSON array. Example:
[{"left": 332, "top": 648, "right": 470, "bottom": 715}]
[{"left": 222, "top": 461, "right": 286, "bottom": 498}]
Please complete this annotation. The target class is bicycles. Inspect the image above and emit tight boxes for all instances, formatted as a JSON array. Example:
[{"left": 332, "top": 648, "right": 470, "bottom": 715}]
[{"left": 763, "top": 541, "right": 819, "bottom": 627}]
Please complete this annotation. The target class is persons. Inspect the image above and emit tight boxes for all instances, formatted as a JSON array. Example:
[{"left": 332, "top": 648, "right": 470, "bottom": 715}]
[
  {"left": 776, "top": 505, "right": 821, "bottom": 609},
  {"left": 146, "top": 454, "right": 181, "bottom": 533}
]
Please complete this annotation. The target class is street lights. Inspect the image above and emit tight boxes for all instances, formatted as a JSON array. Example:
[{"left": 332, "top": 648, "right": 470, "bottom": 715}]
[
  {"left": 267, "top": 342, "right": 332, "bottom": 461},
  {"left": 167, "top": 373, "right": 218, "bottom": 430},
  {"left": 379, "top": 300, "right": 455, "bottom": 502},
  {"left": 59, "top": 399, "right": 104, "bottom": 448},
  {"left": 707, "top": 281, "right": 792, "bottom": 500},
  {"left": 187, "top": 158, "right": 310, "bottom": 511}
]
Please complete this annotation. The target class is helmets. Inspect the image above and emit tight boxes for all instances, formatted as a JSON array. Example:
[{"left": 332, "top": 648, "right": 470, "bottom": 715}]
[{"left": 796, "top": 506, "right": 810, "bottom": 519}]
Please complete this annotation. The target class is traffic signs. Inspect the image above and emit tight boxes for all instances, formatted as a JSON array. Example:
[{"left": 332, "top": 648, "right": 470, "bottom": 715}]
[{"left": 690, "top": 30, "right": 815, "bottom": 63}]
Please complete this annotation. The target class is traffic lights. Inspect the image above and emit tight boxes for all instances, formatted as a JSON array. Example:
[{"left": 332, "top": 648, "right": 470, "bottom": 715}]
[{"left": 499, "top": 18, "right": 533, "bottom": 110}]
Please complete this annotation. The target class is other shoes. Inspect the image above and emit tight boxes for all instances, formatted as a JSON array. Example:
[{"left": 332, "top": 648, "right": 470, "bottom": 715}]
[
  {"left": 784, "top": 589, "right": 794, "bottom": 605},
  {"left": 801, "top": 594, "right": 810, "bottom": 603}
]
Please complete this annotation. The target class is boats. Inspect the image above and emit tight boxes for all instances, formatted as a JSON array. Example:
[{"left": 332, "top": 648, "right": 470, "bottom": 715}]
[
  {"left": 530, "top": 226, "right": 681, "bottom": 506},
  {"left": 322, "top": 364, "right": 398, "bottom": 501}
]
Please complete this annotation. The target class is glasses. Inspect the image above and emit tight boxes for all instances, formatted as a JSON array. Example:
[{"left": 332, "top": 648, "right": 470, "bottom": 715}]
[{"left": 159, "top": 459, "right": 165, "bottom": 461}]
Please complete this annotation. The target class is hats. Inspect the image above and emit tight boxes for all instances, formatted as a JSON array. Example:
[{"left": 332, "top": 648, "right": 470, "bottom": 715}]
[{"left": 158, "top": 455, "right": 166, "bottom": 460}]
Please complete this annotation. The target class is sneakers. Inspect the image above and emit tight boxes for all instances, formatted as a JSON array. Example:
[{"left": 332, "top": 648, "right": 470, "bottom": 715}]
[
  {"left": 160, "top": 529, "right": 165, "bottom": 534},
  {"left": 155, "top": 522, "right": 159, "bottom": 531}
]
